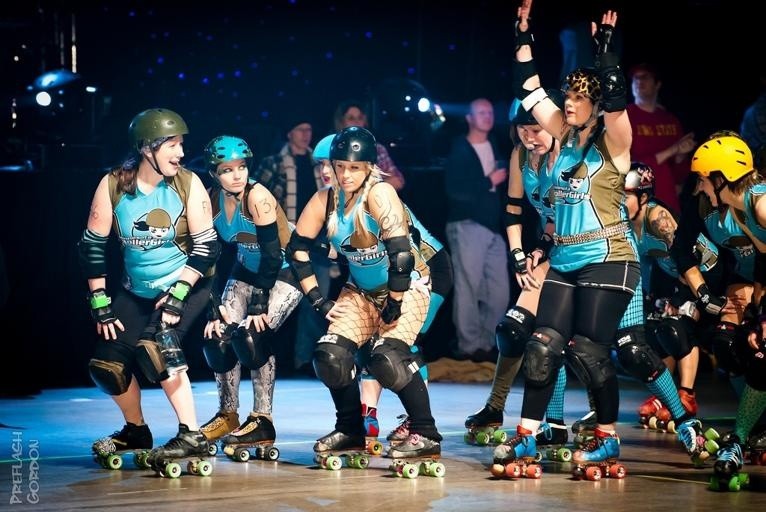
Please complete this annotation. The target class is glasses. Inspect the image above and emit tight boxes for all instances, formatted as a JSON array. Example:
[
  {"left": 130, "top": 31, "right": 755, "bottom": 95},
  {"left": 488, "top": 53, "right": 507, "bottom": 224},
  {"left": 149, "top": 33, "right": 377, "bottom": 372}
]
[
  {"left": 342, "top": 113, "right": 367, "bottom": 122},
  {"left": 295, "top": 127, "right": 311, "bottom": 132}
]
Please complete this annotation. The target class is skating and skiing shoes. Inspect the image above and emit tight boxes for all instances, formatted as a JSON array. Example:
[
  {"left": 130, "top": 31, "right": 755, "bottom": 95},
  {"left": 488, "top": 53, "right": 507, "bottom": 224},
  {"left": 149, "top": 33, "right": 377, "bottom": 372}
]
[
  {"left": 147, "top": 424, "right": 213, "bottom": 479},
  {"left": 387, "top": 434, "right": 446, "bottom": 478},
  {"left": 385, "top": 413, "right": 410, "bottom": 454},
  {"left": 220, "top": 413, "right": 280, "bottom": 462},
  {"left": 748, "top": 430, "right": 766, "bottom": 465},
  {"left": 649, "top": 389, "right": 698, "bottom": 434},
  {"left": 639, "top": 395, "right": 664, "bottom": 429},
  {"left": 463, "top": 403, "right": 507, "bottom": 446},
  {"left": 92, "top": 424, "right": 153, "bottom": 470},
  {"left": 708, "top": 433, "right": 750, "bottom": 491},
  {"left": 571, "top": 410, "right": 598, "bottom": 445},
  {"left": 312, "top": 429, "right": 370, "bottom": 471},
  {"left": 677, "top": 412, "right": 720, "bottom": 467},
  {"left": 535, "top": 421, "right": 571, "bottom": 462},
  {"left": 571, "top": 430, "right": 626, "bottom": 480},
  {"left": 361, "top": 403, "right": 383, "bottom": 456},
  {"left": 492, "top": 424, "right": 542, "bottom": 479},
  {"left": 199, "top": 411, "right": 241, "bottom": 456}
]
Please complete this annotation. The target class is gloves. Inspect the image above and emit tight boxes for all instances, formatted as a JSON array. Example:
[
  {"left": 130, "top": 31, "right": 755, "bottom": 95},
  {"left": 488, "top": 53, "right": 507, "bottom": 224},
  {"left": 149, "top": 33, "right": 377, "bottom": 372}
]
[
  {"left": 509, "top": 14, "right": 535, "bottom": 46},
  {"left": 160, "top": 281, "right": 193, "bottom": 315},
  {"left": 244, "top": 287, "right": 269, "bottom": 318},
  {"left": 88, "top": 288, "right": 118, "bottom": 324},
  {"left": 305, "top": 285, "right": 335, "bottom": 319},
  {"left": 510, "top": 247, "right": 532, "bottom": 274},
  {"left": 696, "top": 285, "right": 728, "bottom": 317},
  {"left": 591, "top": 22, "right": 614, "bottom": 51},
  {"left": 207, "top": 292, "right": 223, "bottom": 320},
  {"left": 527, "top": 234, "right": 554, "bottom": 263},
  {"left": 382, "top": 294, "right": 403, "bottom": 323},
  {"left": 748, "top": 295, "right": 766, "bottom": 339}
]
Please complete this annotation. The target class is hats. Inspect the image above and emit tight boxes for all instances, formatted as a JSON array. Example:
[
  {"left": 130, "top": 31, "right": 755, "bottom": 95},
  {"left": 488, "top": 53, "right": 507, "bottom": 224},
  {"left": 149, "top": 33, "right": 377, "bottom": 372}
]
[{"left": 632, "top": 63, "right": 660, "bottom": 80}]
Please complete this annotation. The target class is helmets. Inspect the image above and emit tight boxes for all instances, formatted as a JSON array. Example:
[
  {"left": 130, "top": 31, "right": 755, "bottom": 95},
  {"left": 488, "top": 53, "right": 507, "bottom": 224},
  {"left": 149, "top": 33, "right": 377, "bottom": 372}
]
[
  {"left": 688, "top": 137, "right": 755, "bottom": 182},
  {"left": 623, "top": 161, "right": 655, "bottom": 190},
  {"left": 710, "top": 129, "right": 740, "bottom": 137},
  {"left": 518, "top": 88, "right": 564, "bottom": 124},
  {"left": 204, "top": 135, "right": 254, "bottom": 165},
  {"left": 509, "top": 97, "right": 523, "bottom": 120},
  {"left": 128, "top": 108, "right": 189, "bottom": 141},
  {"left": 313, "top": 135, "right": 337, "bottom": 159},
  {"left": 562, "top": 67, "right": 606, "bottom": 100},
  {"left": 330, "top": 126, "right": 377, "bottom": 163}
]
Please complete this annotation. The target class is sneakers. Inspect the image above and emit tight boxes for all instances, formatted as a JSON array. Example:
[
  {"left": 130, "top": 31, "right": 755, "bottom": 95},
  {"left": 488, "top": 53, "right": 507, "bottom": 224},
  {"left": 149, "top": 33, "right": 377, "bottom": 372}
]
[
  {"left": 455, "top": 349, "right": 487, "bottom": 362},
  {"left": 489, "top": 345, "right": 500, "bottom": 363}
]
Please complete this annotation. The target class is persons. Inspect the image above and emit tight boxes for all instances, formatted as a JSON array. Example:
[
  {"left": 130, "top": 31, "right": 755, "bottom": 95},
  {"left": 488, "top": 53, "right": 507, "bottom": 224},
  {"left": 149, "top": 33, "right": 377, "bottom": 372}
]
[
  {"left": 254, "top": 123, "right": 330, "bottom": 376},
  {"left": 694, "top": 136, "right": 766, "bottom": 491},
  {"left": 738, "top": 96, "right": 766, "bottom": 178},
  {"left": 467, "top": 103, "right": 569, "bottom": 444},
  {"left": 79, "top": 109, "right": 219, "bottom": 478},
  {"left": 289, "top": 127, "right": 445, "bottom": 477},
  {"left": 335, "top": 103, "right": 406, "bottom": 195},
  {"left": 624, "top": 162, "right": 720, "bottom": 434},
  {"left": 310, "top": 133, "right": 452, "bottom": 453},
  {"left": 626, "top": 67, "right": 698, "bottom": 229},
  {"left": 444, "top": 99, "right": 510, "bottom": 362},
  {"left": 492, "top": 1, "right": 640, "bottom": 479},
  {"left": 202, "top": 137, "right": 306, "bottom": 461}
]
